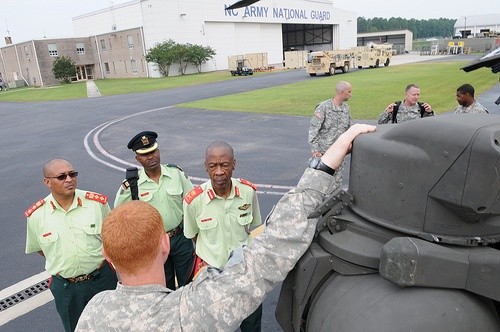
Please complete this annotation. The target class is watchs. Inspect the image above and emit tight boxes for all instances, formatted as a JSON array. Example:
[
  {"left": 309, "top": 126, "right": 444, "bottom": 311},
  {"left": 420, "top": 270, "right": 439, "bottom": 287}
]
[{"left": 308, "top": 155, "right": 335, "bottom": 176}]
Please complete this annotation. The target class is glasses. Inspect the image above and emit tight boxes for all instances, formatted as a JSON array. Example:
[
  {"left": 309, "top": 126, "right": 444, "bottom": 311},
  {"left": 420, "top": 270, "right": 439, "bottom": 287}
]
[{"left": 45, "top": 171, "right": 78, "bottom": 181}]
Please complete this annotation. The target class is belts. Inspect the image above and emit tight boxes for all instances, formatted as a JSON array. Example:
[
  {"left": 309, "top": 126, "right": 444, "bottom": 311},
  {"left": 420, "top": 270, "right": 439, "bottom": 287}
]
[
  {"left": 52, "top": 264, "right": 106, "bottom": 284},
  {"left": 167, "top": 220, "right": 183, "bottom": 240}
]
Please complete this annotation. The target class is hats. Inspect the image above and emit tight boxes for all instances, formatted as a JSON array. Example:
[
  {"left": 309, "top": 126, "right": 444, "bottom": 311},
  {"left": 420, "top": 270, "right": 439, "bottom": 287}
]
[{"left": 127, "top": 130, "right": 158, "bottom": 154}]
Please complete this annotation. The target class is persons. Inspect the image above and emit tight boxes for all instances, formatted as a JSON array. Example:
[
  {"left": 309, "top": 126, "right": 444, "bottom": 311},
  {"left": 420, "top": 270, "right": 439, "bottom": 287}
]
[
  {"left": 454, "top": 84, "right": 489, "bottom": 113},
  {"left": 378, "top": 85, "right": 435, "bottom": 125},
  {"left": 25, "top": 159, "right": 118, "bottom": 332},
  {"left": 74, "top": 123, "right": 375, "bottom": 332},
  {"left": 182, "top": 141, "right": 262, "bottom": 332},
  {"left": 307, "top": 80, "right": 352, "bottom": 188},
  {"left": 113, "top": 131, "right": 195, "bottom": 291}
]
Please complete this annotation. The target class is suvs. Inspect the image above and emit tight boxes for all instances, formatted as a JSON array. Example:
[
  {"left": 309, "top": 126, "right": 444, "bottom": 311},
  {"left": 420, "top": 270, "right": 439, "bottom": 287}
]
[
  {"left": 352, "top": 42, "right": 393, "bottom": 69},
  {"left": 307, "top": 50, "right": 351, "bottom": 77}
]
